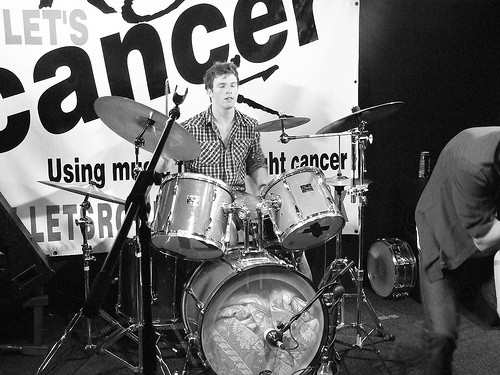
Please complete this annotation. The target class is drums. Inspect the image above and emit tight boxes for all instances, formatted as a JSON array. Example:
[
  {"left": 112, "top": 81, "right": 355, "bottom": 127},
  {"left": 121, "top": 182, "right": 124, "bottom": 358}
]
[
  {"left": 261, "top": 167, "right": 347, "bottom": 249},
  {"left": 368, "top": 238, "right": 416, "bottom": 297},
  {"left": 152, "top": 173, "right": 234, "bottom": 260},
  {"left": 182, "top": 250, "right": 328, "bottom": 375}
]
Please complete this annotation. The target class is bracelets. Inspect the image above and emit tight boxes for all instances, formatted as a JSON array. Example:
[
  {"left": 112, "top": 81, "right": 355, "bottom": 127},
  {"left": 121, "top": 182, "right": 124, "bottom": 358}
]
[{"left": 259, "top": 184, "right": 266, "bottom": 190}]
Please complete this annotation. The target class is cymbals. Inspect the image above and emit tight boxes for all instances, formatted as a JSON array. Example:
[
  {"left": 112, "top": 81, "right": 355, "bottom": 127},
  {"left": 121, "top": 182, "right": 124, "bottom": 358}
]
[
  {"left": 326, "top": 174, "right": 374, "bottom": 187},
  {"left": 253, "top": 116, "right": 311, "bottom": 131},
  {"left": 38, "top": 177, "right": 125, "bottom": 206},
  {"left": 93, "top": 97, "right": 200, "bottom": 161},
  {"left": 315, "top": 102, "right": 405, "bottom": 134}
]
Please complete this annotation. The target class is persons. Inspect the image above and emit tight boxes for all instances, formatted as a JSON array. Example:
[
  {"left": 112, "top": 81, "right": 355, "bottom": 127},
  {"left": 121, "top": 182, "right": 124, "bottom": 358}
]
[
  {"left": 154, "top": 62, "right": 313, "bottom": 297},
  {"left": 415, "top": 126, "right": 500, "bottom": 375}
]
